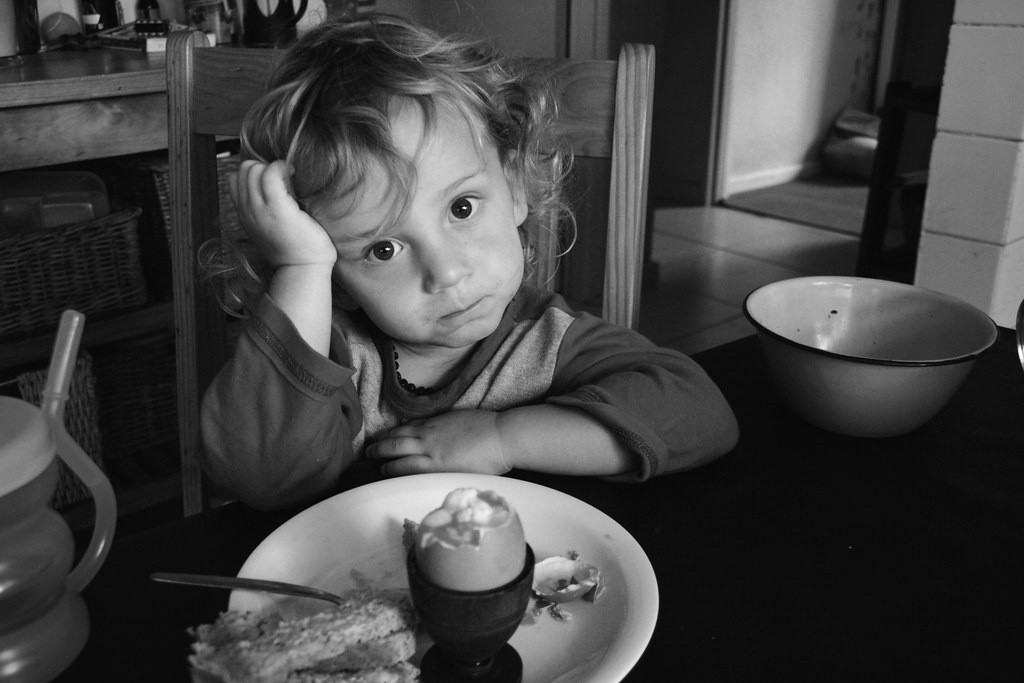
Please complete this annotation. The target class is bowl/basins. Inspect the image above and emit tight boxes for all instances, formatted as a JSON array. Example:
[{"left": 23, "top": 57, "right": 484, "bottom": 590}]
[{"left": 742, "top": 274, "right": 998, "bottom": 440}]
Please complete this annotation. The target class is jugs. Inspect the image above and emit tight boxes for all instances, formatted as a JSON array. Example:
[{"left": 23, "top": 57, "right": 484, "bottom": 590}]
[{"left": 0, "top": 309, "right": 117, "bottom": 681}]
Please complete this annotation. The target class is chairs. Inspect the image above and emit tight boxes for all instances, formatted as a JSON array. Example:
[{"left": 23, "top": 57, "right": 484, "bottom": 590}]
[{"left": 165, "top": 29, "right": 656, "bottom": 518}]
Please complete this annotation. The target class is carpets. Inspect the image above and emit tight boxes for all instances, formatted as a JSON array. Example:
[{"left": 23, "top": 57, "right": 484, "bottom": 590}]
[{"left": 718, "top": 174, "right": 868, "bottom": 237}]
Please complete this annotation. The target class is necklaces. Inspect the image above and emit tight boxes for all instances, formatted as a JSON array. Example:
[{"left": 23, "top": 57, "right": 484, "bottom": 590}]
[{"left": 393, "top": 346, "right": 440, "bottom": 394}]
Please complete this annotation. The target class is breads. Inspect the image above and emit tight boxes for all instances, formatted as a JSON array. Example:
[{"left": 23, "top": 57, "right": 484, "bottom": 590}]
[{"left": 187, "top": 584, "right": 427, "bottom": 683}]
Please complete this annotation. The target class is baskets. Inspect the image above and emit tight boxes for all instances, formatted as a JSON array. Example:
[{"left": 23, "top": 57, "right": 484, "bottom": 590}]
[
  {"left": 150, "top": 150, "right": 250, "bottom": 253},
  {"left": 0, "top": 207, "right": 149, "bottom": 337},
  {"left": 87, "top": 318, "right": 244, "bottom": 458},
  {"left": 0, "top": 355, "right": 105, "bottom": 506}
]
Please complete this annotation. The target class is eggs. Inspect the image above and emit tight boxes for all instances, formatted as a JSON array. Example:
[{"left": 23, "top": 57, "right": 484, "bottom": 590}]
[{"left": 415, "top": 485, "right": 526, "bottom": 593}]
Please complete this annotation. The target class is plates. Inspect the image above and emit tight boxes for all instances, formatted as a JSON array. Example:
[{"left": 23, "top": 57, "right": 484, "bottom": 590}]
[{"left": 227, "top": 471, "right": 660, "bottom": 683}]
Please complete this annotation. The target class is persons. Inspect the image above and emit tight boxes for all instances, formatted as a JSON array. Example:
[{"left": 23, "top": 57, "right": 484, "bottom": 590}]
[{"left": 196, "top": 11, "right": 740, "bottom": 506}]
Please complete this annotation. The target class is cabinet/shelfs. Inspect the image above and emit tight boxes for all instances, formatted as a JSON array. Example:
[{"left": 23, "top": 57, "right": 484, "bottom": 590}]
[{"left": 1, "top": 44, "right": 294, "bottom": 533}]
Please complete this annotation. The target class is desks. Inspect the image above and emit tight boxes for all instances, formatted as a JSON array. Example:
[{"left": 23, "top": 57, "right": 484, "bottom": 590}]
[{"left": 48, "top": 325, "right": 1024, "bottom": 683}]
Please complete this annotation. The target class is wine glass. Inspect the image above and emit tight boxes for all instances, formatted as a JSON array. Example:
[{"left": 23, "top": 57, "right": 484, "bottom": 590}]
[{"left": 405, "top": 541, "right": 535, "bottom": 683}]
